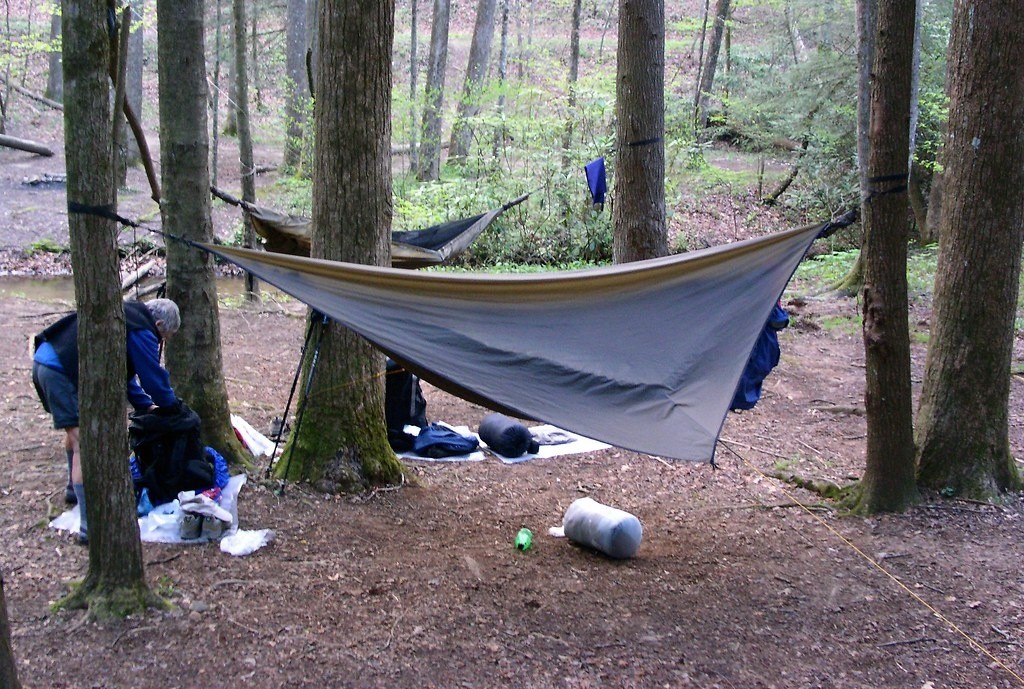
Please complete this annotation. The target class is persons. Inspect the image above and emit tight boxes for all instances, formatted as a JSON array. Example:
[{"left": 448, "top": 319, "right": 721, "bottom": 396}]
[{"left": 32, "top": 298, "right": 180, "bottom": 544}]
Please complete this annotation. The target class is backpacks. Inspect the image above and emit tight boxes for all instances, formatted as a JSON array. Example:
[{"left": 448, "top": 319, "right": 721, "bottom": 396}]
[
  {"left": 386, "top": 359, "right": 428, "bottom": 453},
  {"left": 127, "top": 400, "right": 215, "bottom": 508}
]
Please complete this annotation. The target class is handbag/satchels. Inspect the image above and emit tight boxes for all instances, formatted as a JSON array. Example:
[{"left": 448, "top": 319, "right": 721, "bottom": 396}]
[{"left": 413, "top": 422, "right": 492, "bottom": 458}]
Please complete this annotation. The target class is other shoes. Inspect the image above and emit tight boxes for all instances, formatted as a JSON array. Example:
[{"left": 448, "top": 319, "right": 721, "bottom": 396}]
[
  {"left": 64, "top": 485, "right": 77, "bottom": 504},
  {"left": 78, "top": 526, "right": 88, "bottom": 544}
]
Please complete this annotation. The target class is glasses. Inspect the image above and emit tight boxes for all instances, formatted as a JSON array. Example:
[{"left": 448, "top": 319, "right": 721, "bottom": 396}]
[{"left": 162, "top": 328, "right": 167, "bottom": 341}]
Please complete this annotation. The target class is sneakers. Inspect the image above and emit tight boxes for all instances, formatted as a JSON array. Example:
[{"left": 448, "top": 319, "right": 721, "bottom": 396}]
[
  {"left": 202, "top": 515, "right": 225, "bottom": 539},
  {"left": 180, "top": 514, "right": 203, "bottom": 539}
]
